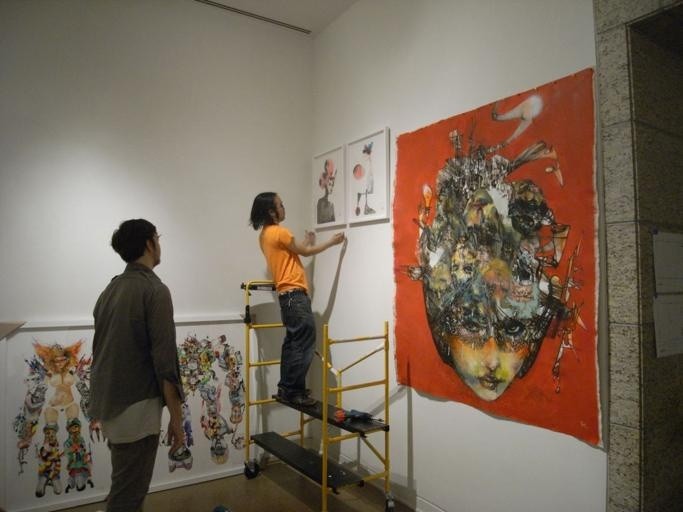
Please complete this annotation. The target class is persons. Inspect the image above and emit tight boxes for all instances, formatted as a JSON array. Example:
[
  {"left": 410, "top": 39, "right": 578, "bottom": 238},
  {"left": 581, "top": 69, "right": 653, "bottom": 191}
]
[
  {"left": 246, "top": 190, "right": 346, "bottom": 407},
  {"left": 316, "top": 162, "right": 338, "bottom": 222},
  {"left": 87, "top": 217, "right": 187, "bottom": 511},
  {"left": 411, "top": 130, "right": 565, "bottom": 402}
]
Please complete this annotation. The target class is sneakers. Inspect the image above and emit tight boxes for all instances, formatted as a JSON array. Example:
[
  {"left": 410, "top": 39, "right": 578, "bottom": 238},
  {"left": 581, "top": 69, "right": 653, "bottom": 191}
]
[{"left": 277, "top": 388, "right": 318, "bottom": 408}]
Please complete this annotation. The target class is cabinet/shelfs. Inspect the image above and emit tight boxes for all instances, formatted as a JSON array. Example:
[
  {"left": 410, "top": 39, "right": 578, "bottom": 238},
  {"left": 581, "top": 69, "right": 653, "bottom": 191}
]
[{"left": 239, "top": 278, "right": 400, "bottom": 511}]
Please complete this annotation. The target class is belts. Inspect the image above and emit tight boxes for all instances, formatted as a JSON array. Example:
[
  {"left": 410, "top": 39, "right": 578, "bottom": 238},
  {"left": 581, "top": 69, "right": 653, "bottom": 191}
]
[{"left": 277, "top": 286, "right": 305, "bottom": 299}]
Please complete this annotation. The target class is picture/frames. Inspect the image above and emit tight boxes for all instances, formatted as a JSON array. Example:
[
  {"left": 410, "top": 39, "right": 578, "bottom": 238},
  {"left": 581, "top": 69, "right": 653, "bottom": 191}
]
[
  {"left": 0, "top": 313, "right": 263, "bottom": 511},
  {"left": 312, "top": 125, "right": 393, "bottom": 230}
]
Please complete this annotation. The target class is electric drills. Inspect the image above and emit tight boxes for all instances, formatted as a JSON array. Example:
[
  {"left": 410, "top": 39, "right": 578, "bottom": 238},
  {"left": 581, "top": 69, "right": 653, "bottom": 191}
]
[{"left": 333, "top": 409, "right": 384, "bottom": 423}]
